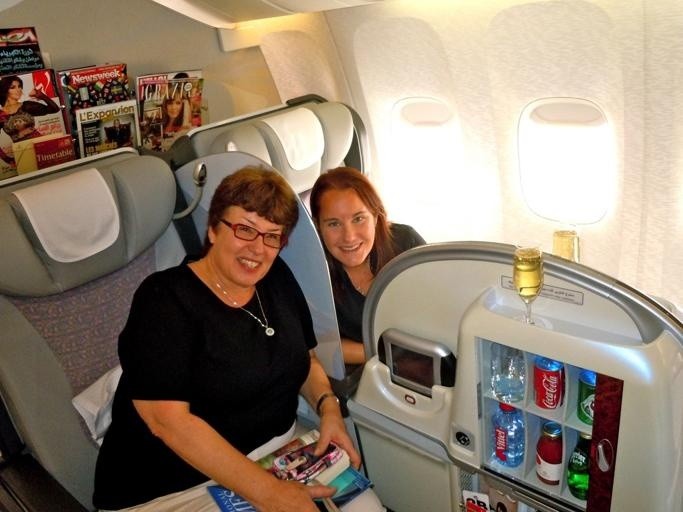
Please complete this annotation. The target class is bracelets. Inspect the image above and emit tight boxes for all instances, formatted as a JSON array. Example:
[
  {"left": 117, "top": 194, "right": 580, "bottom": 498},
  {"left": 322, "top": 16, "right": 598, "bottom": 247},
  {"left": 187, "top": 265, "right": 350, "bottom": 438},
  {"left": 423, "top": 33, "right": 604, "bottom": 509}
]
[{"left": 316, "top": 391, "right": 341, "bottom": 417}]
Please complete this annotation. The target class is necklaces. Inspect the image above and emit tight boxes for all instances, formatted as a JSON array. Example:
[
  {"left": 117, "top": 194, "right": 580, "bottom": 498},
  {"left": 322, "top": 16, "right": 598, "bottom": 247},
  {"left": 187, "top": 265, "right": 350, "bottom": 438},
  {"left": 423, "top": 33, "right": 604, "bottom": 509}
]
[
  {"left": 204, "top": 264, "right": 276, "bottom": 336},
  {"left": 355, "top": 266, "right": 370, "bottom": 295}
]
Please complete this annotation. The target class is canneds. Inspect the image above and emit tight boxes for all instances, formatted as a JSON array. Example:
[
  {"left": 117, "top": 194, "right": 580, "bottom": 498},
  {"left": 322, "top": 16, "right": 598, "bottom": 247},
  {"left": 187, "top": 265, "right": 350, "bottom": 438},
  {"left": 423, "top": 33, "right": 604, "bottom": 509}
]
[
  {"left": 534, "top": 356, "right": 564, "bottom": 409},
  {"left": 578, "top": 371, "right": 596, "bottom": 425}
]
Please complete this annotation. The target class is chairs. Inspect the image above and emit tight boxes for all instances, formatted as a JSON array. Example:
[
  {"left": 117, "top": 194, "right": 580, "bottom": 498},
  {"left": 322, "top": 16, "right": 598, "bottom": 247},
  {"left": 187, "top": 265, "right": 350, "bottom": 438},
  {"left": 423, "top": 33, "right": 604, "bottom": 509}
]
[
  {"left": 0, "top": 147, "right": 196, "bottom": 512},
  {"left": 168, "top": 94, "right": 371, "bottom": 481}
]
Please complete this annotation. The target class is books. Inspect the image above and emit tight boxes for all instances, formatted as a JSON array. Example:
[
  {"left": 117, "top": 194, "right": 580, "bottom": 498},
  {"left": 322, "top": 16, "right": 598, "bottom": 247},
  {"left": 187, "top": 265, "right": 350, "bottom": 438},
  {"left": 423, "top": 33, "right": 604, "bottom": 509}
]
[
  {"left": 207, "top": 429, "right": 372, "bottom": 512},
  {"left": 0, "top": 26, "right": 210, "bottom": 181}
]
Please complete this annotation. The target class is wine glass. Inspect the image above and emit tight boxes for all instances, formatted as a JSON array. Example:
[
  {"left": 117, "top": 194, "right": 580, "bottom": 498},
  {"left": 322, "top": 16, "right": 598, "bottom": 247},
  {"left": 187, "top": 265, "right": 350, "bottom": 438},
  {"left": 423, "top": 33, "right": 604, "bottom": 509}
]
[
  {"left": 510, "top": 245, "right": 547, "bottom": 331},
  {"left": 552, "top": 230, "right": 581, "bottom": 264}
]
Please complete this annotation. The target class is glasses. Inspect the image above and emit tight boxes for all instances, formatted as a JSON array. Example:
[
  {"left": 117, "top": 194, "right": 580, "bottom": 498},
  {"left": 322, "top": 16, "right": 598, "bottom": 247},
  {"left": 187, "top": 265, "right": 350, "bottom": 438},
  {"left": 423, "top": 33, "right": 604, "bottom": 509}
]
[{"left": 214, "top": 215, "right": 288, "bottom": 250}]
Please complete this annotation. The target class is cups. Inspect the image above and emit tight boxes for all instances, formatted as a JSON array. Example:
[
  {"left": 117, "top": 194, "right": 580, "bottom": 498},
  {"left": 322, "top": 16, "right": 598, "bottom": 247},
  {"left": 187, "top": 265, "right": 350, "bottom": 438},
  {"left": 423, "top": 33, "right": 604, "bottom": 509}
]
[{"left": 488, "top": 341, "right": 525, "bottom": 404}]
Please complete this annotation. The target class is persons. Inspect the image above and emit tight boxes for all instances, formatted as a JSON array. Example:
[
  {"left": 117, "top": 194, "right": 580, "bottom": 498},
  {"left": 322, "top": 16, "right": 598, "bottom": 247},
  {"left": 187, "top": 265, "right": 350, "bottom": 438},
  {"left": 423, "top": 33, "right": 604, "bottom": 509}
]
[
  {"left": 92, "top": 165, "right": 384, "bottom": 511},
  {"left": 311, "top": 164, "right": 427, "bottom": 417}
]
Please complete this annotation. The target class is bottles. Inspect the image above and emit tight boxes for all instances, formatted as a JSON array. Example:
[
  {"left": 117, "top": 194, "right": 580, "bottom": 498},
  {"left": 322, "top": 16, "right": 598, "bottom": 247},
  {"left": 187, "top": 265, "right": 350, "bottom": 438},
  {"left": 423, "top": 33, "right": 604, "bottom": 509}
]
[
  {"left": 566, "top": 432, "right": 592, "bottom": 499},
  {"left": 533, "top": 420, "right": 564, "bottom": 486},
  {"left": 490, "top": 400, "right": 525, "bottom": 467}
]
